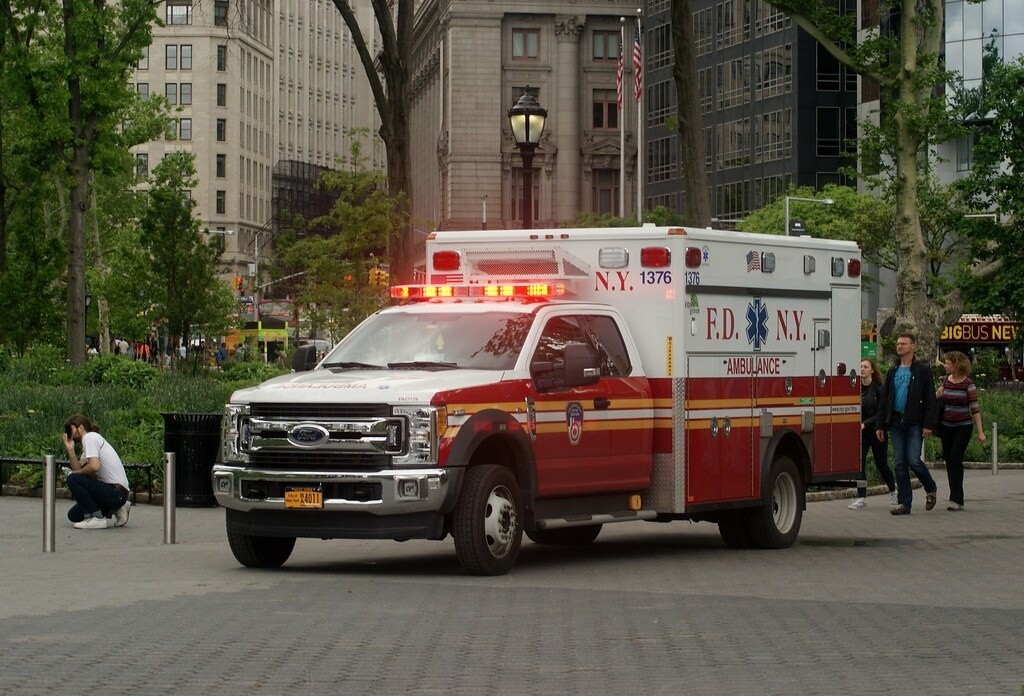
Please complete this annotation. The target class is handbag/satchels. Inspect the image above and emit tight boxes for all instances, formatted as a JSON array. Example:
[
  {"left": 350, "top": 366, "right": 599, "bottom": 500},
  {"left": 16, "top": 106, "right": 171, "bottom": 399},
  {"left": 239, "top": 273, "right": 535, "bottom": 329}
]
[{"left": 931, "top": 376, "right": 946, "bottom": 438}]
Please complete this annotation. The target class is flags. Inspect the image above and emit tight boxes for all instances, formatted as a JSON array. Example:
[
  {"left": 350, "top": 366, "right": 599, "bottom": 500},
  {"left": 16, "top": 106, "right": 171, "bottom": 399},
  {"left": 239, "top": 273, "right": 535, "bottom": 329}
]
[
  {"left": 632, "top": 20, "right": 644, "bottom": 102},
  {"left": 616, "top": 41, "right": 625, "bottom": 110}
]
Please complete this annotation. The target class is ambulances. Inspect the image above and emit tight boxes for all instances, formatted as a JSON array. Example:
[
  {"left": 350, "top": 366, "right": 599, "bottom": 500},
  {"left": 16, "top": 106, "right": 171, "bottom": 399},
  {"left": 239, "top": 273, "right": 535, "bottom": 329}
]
[{"left": 212, "top": 222, "right": 869, "bottom": 576}]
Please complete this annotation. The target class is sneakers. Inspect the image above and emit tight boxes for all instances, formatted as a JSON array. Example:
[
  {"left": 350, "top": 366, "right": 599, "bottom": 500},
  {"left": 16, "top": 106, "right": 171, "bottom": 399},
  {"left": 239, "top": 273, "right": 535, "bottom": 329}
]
[
  {"left": 947, "top": 501, "right": 964, "bottom": 510},
  {"left": 890, "top": 503, "right": 910, "bottom": 515},
  {"left": 73, "top": 517, "right": 108, "bottom": 529},
  {"left": 848, "top": 498, "right": 868, "bottom": 510},
  {"left": 888, "top": 490, "right": 898, "bottom": 508},
  {"left": 926, "top": 488, "right": 937, "bottom": 509},
  {"left": 114, "top": 501, "right": 131, "bottom": 526}
]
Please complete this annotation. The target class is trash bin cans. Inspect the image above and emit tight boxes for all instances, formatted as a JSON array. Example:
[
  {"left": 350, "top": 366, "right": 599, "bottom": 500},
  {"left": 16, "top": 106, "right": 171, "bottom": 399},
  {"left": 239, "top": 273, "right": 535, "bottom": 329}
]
[{"left": 158, "top": 411, "right": 226, "bottom": 509}]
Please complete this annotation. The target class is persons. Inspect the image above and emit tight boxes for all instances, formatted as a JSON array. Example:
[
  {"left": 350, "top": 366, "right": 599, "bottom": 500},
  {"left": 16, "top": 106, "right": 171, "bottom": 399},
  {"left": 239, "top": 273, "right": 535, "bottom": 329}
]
[
  {"left": 87, "top": 336, "right": 248, "bottom": 371},
  {"left": 848, "top": 335, "right": 986, "bottom": 515},
  {"left": 60, "top": 414, "right": 132, "bottom": 529}
]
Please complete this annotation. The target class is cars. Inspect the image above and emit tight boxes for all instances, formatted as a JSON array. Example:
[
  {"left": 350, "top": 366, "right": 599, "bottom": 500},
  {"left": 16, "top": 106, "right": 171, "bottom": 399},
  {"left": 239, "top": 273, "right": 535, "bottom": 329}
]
[{"left": 293, "top": 338, "right": 335, "bottom": 361}]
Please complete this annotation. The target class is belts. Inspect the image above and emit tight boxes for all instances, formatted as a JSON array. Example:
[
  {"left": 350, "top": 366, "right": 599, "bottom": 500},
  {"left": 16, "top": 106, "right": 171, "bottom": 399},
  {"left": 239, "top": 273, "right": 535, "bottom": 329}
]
[
  {"left": 112, "top": 484, "right": 129, "bottom": 493},
  {"left": 892, "top": 412, "right": 903, "bottom": 417}
]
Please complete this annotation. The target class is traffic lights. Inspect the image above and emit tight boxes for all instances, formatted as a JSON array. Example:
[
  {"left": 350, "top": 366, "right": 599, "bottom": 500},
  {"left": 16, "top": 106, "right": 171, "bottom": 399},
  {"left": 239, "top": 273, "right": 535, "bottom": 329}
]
[
  {"left": 382, "top": 271, "right": 389, "bottom": 288},
  {"left": 368, "top": 268, "right": 375, "bottom": 286},
  {"left": 373, "top": 269, "right": 382, "bottom": 287}
]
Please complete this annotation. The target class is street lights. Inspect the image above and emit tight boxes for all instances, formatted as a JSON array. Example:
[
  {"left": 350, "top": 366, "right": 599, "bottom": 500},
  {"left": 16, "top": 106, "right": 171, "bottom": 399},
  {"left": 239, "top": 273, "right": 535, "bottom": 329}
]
[
  {"left": 507, "top": 82, "right": 550, "bottom": 229},
  {"left": 784, "top": 196, "right": 835, "bottom": 235}
]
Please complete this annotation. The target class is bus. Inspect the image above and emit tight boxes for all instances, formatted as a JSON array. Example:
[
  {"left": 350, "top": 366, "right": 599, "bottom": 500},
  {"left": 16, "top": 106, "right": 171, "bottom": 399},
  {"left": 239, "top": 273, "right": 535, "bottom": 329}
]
[{"left": 223, "top": 298, "right": 299, "bottom": 368}]
